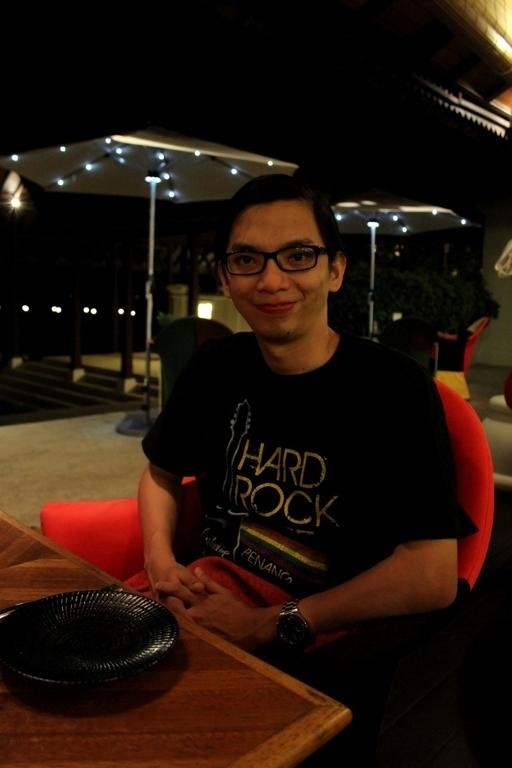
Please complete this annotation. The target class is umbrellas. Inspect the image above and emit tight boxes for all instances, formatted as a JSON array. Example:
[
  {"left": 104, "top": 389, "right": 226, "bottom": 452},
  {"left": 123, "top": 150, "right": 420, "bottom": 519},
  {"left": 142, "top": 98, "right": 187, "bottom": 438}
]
[
  {"left": 1, "top": 129, "right": 300, "bottom": 424},
  {"left": 327, "top": 190, "right": 480, "bottom": 336}
]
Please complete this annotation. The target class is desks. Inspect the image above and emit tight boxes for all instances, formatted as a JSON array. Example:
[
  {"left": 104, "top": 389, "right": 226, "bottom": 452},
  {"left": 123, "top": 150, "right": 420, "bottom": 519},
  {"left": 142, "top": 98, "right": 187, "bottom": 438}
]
[{"left": 0, "top": 510, "right": 351, "bottom": 767}]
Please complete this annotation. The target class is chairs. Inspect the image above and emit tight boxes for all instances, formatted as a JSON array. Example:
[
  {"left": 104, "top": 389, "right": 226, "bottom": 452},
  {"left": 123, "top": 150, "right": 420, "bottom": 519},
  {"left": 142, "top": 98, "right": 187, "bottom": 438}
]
[{"left": 41, "top": 384, "right": 494, "bottom": 653}]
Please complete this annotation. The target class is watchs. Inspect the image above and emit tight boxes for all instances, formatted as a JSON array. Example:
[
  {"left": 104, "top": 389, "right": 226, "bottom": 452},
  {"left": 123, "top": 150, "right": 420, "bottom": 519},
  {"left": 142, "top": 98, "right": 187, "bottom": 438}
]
[{"left": 278, "top": 599, "right": 313, "bottom": 649}]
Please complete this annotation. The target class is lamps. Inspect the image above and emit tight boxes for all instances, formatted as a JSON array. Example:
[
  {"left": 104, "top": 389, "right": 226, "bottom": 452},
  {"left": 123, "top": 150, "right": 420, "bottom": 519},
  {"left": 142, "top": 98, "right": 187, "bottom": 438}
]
[
  {"left": 4, "top": 139, "right": 276, "bottom": 216},
  {"left": 329, "top": 194, "right": 487, "bottom": 242}
]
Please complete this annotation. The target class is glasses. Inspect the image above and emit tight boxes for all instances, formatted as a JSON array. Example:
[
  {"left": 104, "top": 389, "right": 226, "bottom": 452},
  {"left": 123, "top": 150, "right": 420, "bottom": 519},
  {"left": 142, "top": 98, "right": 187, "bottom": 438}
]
[{"left": 219, "top": 244, "right": 330, "bottom": 276}]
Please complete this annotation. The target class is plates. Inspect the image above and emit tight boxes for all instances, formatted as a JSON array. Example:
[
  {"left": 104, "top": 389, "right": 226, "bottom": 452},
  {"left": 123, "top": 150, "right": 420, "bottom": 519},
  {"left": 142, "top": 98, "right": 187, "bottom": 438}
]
[{"left": 0, "top": 589, "right": 180, "bottom": 687}]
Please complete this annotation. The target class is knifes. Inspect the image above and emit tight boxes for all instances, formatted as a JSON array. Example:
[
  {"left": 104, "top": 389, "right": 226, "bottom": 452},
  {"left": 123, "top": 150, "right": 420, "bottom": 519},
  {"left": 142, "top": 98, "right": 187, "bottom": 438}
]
[{"left": 0, "top": 582, "right": 124, "bottom": 621}]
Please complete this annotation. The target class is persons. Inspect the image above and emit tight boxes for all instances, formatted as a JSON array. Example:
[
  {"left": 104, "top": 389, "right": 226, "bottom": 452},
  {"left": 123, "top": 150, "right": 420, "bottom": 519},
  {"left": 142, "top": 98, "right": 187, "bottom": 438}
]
[{"left": 126, "top": 175, "right": 481, "bottom": 656}]
